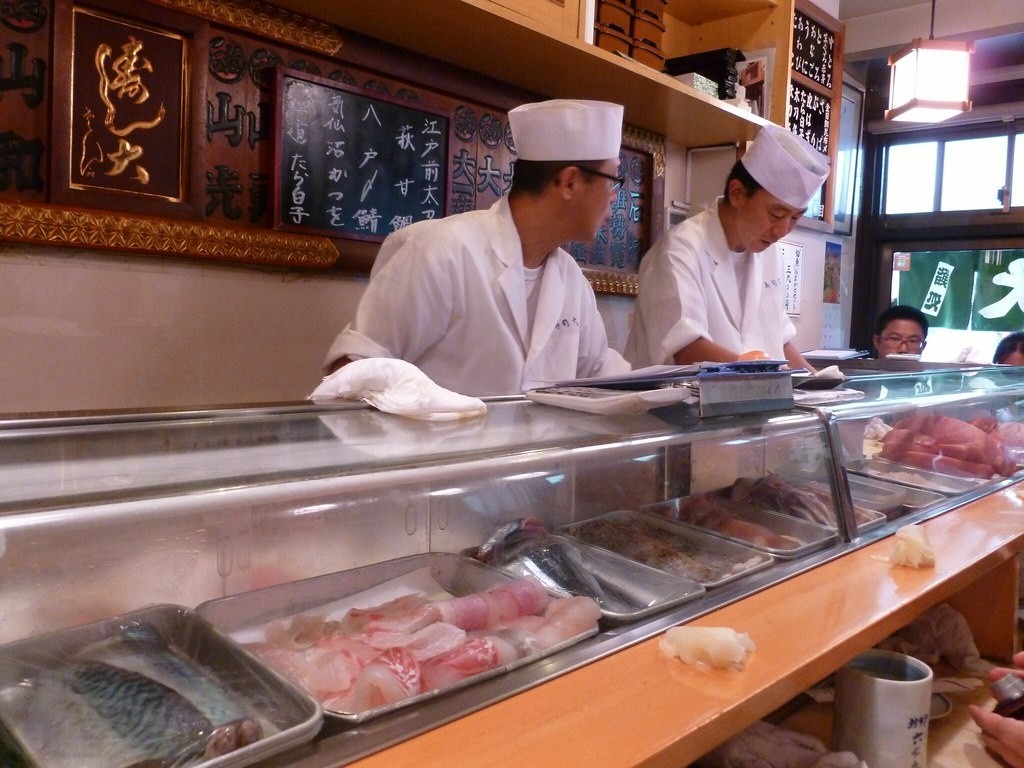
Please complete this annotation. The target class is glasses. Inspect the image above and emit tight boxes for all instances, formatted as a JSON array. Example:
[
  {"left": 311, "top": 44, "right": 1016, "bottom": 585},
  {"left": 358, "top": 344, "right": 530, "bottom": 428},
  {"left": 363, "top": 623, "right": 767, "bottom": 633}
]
[
  {"left": 877, "top": 336, "right": 927, "bottom": 352},
  {"left": 560, "top": 164, "right": 626, "bottom": 192}
]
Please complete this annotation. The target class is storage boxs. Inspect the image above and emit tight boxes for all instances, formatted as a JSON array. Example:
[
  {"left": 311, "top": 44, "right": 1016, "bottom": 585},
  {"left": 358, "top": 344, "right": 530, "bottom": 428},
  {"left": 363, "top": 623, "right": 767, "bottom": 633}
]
[
  {"left": 629, "top": 10, "right": 666, "bottom": 51},
  {"left": 629, "top": 39, "right": 665, "bottom": 72},
  {"left": 598, "top": 0, "right": 634, "bottom": 37},
  {"left": 596, "top": 26, "right": 634, "bottom": 57},
  {"left": 633, "top": 0, "right": 669, "bottom": 23}
]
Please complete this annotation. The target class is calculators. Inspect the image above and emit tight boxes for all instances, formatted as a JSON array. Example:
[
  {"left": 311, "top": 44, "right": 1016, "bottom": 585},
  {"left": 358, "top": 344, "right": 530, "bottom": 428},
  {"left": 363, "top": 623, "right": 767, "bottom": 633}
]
[{"left": 523, "top": 384, "right": 692, "bottom": 418}]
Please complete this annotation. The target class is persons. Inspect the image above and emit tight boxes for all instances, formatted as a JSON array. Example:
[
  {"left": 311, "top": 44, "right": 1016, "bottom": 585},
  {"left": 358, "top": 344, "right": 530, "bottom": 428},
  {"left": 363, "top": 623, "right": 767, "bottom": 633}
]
[
  {"left": 319, "top": 97, "right": 626, "bottom": 399},
  {"left": 872, "top": 305, "right": 929, "bottom": 359},
  {"left": 622, "top": 123, "right": 832, "bottom": 373},
  {"left": 992, "top": 331, "right": 1024, "bottom": 365},
  {"left": 967, "top": 651, "right": 1024, "bottom": 768}
]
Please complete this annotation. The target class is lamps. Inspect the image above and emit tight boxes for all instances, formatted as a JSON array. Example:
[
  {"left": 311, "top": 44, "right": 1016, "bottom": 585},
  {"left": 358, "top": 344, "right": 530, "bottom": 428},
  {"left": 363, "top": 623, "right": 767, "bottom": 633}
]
[{"left": 884, "top": 0, "right": 977, "bottom": 123}]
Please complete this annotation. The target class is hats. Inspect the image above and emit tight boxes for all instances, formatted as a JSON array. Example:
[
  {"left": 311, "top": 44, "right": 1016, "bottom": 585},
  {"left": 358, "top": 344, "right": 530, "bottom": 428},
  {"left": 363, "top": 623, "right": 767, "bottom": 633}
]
[
  {"left": 507, "top": 99, "right": 624, "bottom": 161},
  {"left": 741, "top": 124, "right": 831, "bottom": 209}
]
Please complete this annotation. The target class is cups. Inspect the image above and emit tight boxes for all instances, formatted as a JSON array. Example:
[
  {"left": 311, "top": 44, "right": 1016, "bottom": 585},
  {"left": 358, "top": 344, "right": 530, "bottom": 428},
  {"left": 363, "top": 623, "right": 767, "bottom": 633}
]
[{"left": 831, "top": 649, "right": 934, "bottom": 768}]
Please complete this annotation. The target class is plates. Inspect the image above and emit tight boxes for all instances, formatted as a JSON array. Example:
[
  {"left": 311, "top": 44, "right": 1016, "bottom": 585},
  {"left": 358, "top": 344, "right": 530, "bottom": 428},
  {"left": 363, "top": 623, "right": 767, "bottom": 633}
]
[
  {"left": 886, "top": 354, "right": 920, "bottom": 360},
  {"left": 929, "top": 690, "right": 953, "bottom": 719}
]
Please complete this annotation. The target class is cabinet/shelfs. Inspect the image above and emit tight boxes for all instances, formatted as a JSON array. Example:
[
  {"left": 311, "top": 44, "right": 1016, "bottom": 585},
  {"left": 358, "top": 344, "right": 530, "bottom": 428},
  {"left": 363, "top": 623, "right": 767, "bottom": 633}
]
[{"left": 266, "top": 0, "right": 795, "bottom": 146}]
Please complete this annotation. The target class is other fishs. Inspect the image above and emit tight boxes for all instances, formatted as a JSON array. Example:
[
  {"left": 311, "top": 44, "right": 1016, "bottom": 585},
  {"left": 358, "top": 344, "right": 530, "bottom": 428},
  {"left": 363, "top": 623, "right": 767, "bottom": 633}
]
[
  {"left": 0, "top": 624, "right": 294, "bottom": 768},
  {"left": 478, "top": 517, "right": 610, "bottom": 612}
]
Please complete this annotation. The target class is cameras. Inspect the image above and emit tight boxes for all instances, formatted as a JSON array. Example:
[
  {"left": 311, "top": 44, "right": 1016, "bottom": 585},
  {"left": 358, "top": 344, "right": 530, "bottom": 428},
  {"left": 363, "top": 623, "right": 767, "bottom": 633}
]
[{"left": 990, "top": 673, "right": 1024, "bottom": 721}]
